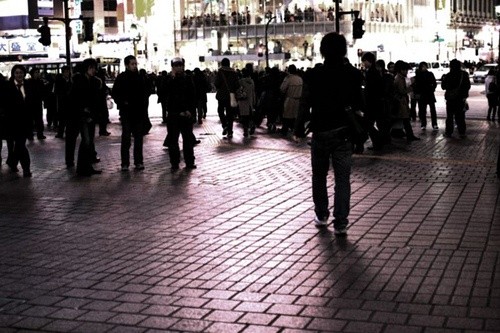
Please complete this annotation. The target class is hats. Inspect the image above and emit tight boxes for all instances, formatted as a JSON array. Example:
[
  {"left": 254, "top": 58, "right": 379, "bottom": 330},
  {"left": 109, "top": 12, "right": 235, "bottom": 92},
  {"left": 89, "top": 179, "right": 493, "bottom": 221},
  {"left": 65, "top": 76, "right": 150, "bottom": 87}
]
[{"left": 171, "top": 57, "right": 184, "bottom": 65}]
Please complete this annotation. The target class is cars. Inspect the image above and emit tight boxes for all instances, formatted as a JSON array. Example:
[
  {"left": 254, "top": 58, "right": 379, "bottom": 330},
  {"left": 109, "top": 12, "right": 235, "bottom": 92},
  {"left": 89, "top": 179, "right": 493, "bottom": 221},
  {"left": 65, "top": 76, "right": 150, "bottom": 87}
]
[{"left": 473, "top": 63, "right": 498, "bottom": 83}]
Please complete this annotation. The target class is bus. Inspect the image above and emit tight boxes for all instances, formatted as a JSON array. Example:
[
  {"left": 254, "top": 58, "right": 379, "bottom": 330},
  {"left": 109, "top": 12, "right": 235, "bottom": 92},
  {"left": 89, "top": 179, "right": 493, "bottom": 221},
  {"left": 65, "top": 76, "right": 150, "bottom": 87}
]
[{"left": 9, "top": 57, "right": 124, "bottom": 89}]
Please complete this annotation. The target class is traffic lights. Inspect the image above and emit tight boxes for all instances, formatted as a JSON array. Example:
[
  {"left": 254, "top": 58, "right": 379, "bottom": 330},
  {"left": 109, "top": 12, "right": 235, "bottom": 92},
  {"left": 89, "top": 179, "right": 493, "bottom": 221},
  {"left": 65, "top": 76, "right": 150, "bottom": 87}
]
[
  {"left": 82, "top": 20, "right": 94, "bottom": 41},
  {"left": 353, "top": 17, "right": 366, "bottom": 39},
  {"left": 38, "top": 25, "right": 51, "bottom": 46}
]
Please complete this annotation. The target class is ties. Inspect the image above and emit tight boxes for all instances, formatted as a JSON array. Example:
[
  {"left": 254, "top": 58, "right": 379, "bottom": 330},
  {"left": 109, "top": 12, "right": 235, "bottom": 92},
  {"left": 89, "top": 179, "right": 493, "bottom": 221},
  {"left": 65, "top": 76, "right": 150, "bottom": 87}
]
[{"left": 17, "top": 83, "right": 22, "bottom": 90}]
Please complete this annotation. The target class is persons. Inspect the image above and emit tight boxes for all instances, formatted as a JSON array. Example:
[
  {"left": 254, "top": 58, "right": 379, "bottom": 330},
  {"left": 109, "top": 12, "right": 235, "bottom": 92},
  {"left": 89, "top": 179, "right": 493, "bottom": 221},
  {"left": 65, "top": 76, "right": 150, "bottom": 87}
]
[
  {"left": 0, "top": 5, "right": 500, "bottom": 177},
  {"left": 295, "top": 32, "right": 365, "bottom": 235}
]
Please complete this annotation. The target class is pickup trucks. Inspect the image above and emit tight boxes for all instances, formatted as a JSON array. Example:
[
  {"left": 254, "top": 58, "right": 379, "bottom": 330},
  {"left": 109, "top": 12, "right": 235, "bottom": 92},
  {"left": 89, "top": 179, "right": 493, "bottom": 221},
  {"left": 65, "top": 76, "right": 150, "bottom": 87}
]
[{"left": 427, "top": 62, "right": 450, "bottom": 81}]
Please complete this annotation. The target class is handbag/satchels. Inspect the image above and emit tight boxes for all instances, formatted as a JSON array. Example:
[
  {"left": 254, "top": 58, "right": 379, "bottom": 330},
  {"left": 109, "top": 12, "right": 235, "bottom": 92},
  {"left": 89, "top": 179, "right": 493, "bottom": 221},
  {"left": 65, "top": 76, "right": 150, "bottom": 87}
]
[
  {"left": 345, "top": 106, "right": 368, "bottom": 146},
  {"left": 230, "top": 92, "right": 238, "bottom": 107},
  {"left": 237, "top": 87, "right": 248, "bottom": 99}
]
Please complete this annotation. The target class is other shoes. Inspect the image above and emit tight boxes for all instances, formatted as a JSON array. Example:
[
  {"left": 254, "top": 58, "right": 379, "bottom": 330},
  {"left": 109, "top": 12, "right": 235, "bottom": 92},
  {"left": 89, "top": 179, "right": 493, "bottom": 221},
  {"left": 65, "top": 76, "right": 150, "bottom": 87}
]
[
  {"left": 492, "top": 118, "right": 495, "bottom": 121},
  {"left": 314, "top": 214, "right": 327, "bottom": 225},
  {"left": 445, "top": 132, "right": 451, "bottom": 137},
  {"left": 421, "top": 126, "right": 425, "bottom": 130},
  {"left": 6, "top": 121, "right": 200, "bottom": 178},
  {"left": 412, "top": 117, "right": 416, "bottom": 120},
  {"left": 433, "top": 125, "right": 438, "bottom": 129},
  {"left": 460, "top": 134, "right": 467, "bottom": 138},
  {"left": 487, "top": 116, "right": 491, "bottom": 121},
  {"left": 222, "top": 119, "right": 312, "bottom": 145},
  {"left": 333, "top": 224, "right": 346, "bottom": 234}
]
